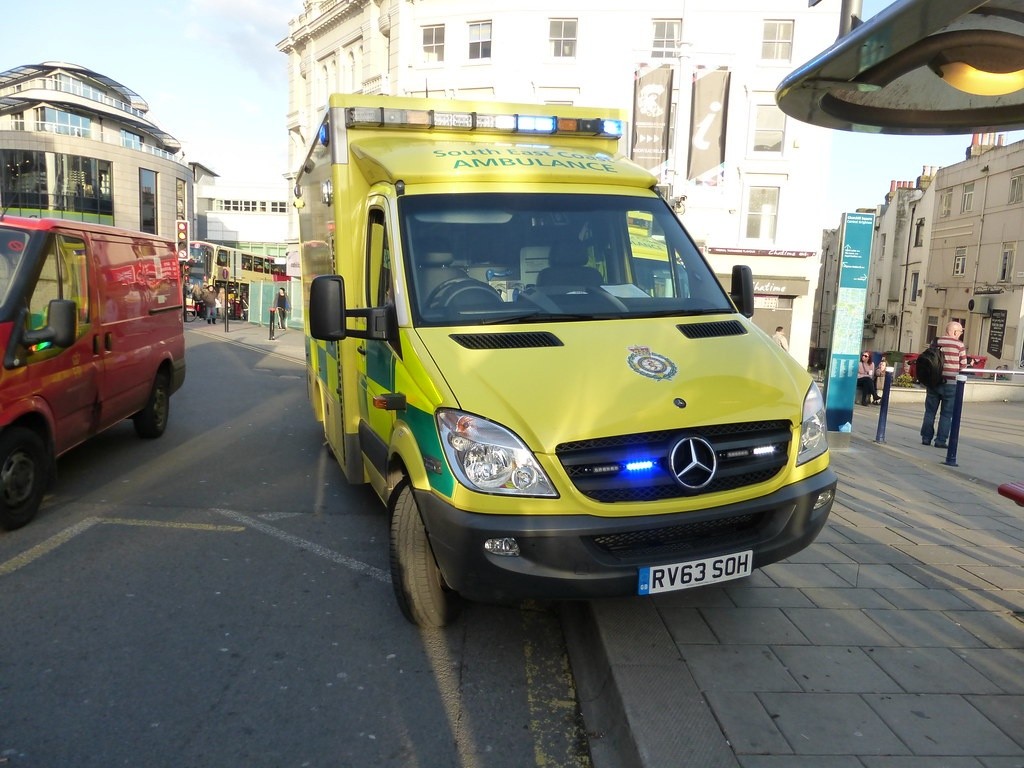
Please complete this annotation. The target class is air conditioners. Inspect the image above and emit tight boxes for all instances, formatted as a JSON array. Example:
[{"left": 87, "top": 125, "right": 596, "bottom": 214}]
[
  {"left": 966, "top": 296, "right": 989, "bottom": 314},
  {"left": 864, "top": 309, "right": 891, "bottom": 325}
]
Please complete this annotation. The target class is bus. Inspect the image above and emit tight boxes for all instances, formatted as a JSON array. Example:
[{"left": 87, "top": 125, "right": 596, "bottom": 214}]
[{"left": 187, "top": 241, "right": 276, "bottom": 316}]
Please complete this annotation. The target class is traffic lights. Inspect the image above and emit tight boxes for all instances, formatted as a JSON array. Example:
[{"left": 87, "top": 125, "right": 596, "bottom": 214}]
[{"left": 175, "top": 219, "right": 191, "bottom": 262}]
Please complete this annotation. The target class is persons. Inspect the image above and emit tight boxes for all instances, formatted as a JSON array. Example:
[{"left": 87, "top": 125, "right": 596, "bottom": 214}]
[
  {"left": 273, "top": 287, "right": 290, "bottom": 331},
  {"left": 875, "top": 361, "right": 888, "bottom": 378},
  {"left": 960, "top": 357, "right": 975, "bottom": 375},
  {"left": 856, "top": 351, "right": 883, "bottom": 407},
  {"left": 190, "top": 281, "right": 231, "bottom": 325},
  {"left": 919, "top": 321, "right": 968, "bottom": 448},
  {"left": 772, "top": 326, "right": 789, "bottom": 352}
]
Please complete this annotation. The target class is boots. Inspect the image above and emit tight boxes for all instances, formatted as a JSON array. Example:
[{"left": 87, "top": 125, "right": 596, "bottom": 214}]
[
  {"left": 212, "top": 318, "right": 216, "bottom": 324},
  {"left": 207, "top": 319, "right": 211, "bottom": 324}
]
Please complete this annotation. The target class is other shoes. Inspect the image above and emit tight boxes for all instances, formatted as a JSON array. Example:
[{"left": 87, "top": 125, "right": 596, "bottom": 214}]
[
  {"left": 862, "top": 401, "right": 867, "bottom": 406},
  {"left": 873, "top": 396, "right": 882, "bottom": 400},
  {"left": 922, "top": 438, "right": 931, "bottom": 445},
  {"left": 934, "top": 442, "right": 948, "bottom": 448},
  {"left": 279, "top": 327, "right": 285, "bottom": 330}
]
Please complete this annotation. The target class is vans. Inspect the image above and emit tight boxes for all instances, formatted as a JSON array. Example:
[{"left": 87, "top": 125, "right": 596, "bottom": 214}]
[{"left": 0, "top": 213, "right": 186, "bottom": 531}]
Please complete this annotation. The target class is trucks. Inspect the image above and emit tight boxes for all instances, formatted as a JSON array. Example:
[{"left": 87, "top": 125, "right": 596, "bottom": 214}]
[{"left": 292, "top": 92, "right": 837, "bottom": 628}]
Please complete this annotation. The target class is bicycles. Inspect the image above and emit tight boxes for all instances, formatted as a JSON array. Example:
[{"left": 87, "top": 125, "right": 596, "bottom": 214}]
[{"left": 185, "top": 306, "right": 197, "bottom": 322}]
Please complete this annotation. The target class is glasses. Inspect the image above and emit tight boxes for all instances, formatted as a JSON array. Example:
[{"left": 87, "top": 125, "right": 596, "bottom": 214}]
[{"left": 861, "top": 354, "right": 869, "bottom": 357}]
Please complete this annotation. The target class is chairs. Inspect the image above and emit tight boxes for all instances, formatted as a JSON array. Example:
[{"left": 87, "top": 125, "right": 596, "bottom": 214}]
[
  {"left": 415, "top": 244, "right": 469, "bottom": 311},
  {"left": 0, "top": 255, "right": 9, "bottom": 304},
  {"left": 537, "top": 236, "right": 604, "bottom": 292},
  {"left": 29, "top": 255, "right": 69, "bottom": 315}
]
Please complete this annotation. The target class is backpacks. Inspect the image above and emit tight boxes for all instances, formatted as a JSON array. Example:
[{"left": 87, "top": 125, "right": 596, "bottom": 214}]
[{"left": 914, "top": 337, "right": 946, "bottom": 390}]
